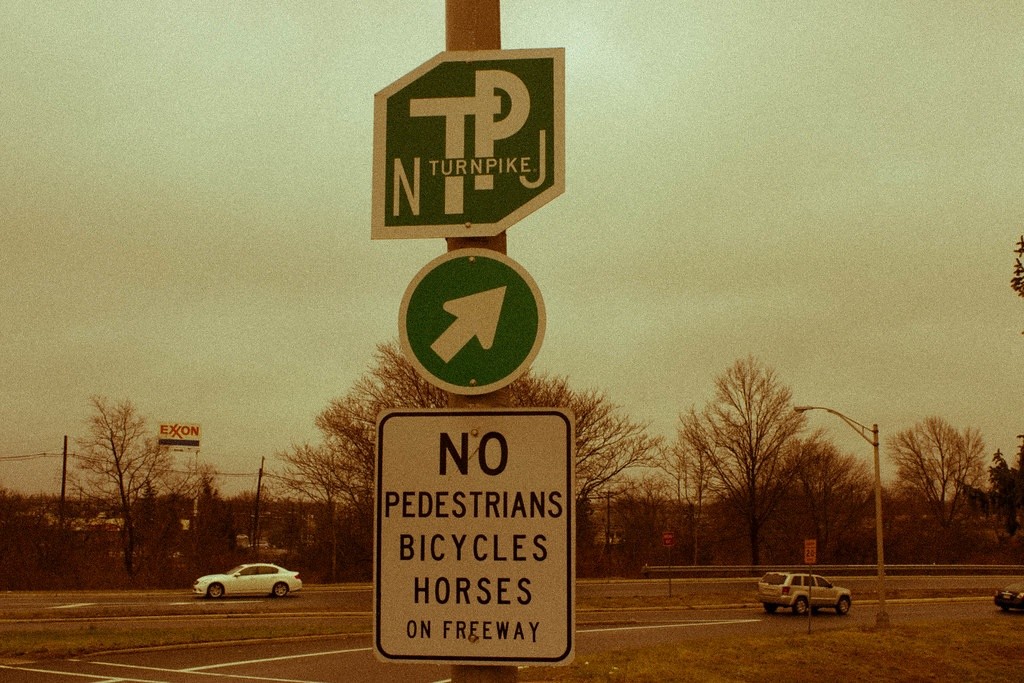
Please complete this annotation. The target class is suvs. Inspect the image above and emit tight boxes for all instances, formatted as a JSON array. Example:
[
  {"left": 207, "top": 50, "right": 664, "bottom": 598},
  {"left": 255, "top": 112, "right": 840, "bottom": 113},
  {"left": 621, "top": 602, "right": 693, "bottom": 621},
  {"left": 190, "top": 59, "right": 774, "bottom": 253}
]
[{"left": 755, "top": 573, "right": 853, "bottom": 615}]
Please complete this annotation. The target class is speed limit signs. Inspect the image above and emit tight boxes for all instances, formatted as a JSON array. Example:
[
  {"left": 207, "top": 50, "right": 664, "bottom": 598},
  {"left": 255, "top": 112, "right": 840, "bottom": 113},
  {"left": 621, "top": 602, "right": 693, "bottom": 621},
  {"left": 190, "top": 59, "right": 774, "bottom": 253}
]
[{"left": 804, "top": 539, "right": 816, "bottom": 563}]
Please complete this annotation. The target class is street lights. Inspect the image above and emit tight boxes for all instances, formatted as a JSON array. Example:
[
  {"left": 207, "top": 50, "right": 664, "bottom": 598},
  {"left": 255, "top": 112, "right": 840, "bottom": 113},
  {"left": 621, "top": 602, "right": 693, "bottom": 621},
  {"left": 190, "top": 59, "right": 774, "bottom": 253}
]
[{"left": 794, "top": 405, "right": 889, "bottom": 625}]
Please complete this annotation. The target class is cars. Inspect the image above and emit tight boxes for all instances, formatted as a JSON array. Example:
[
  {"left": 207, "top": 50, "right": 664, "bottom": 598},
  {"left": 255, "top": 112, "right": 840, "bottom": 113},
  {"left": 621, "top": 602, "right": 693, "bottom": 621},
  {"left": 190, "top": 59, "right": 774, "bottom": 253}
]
[
  {"left": 192, "top": 562, "right": 302, "bottom": 598},
  {"left": 993, "top": 579, "right": 1024, "bottom": 613}
]
